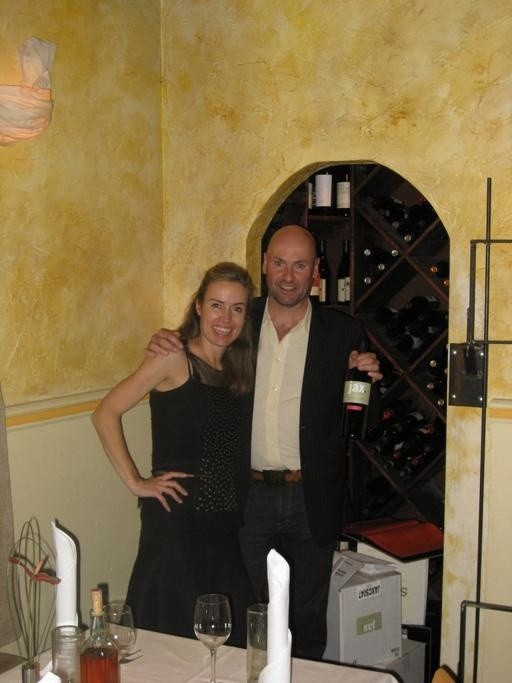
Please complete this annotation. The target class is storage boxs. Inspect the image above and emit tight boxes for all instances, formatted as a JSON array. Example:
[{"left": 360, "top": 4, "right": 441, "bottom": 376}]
[{"left": 331, "top": 538, "right": 433, "bottom": 683}]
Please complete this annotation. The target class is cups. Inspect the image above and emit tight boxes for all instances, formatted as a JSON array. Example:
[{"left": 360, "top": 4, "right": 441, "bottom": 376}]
[
  {"left": 96, "top": 600, "right": 134, "bottom": 665},
  {"left": 246, "top": 601, "right": 268, "bottom": 681},
  {"left": 49, "top": 625, "right": 85, "bottom": 682}
]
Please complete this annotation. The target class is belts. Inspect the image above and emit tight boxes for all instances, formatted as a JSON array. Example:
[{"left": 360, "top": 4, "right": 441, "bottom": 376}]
[{"left": 250, "top": 468, "right": 303, "bottom": 484}]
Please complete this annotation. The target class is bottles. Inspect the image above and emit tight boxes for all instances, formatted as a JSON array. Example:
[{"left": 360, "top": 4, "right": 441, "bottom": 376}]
[
  {"left": 78, "top": 586, "right": 120, "bottom": 682},
  {"left": 314, "top": 165, "right": 451, "bottom": 490}
]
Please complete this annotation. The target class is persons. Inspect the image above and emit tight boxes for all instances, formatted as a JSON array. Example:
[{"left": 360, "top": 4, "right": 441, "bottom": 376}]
[
  {"left": 92, "top": 262, "right": 267, "bottom": 653},
  {"left": 142, "top": 224, "right": 383, "bottom": 661}
]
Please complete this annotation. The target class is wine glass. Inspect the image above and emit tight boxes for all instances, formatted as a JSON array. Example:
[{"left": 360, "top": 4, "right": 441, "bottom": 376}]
[{"left": 193, "top": 593, "right": 232, "bottom": 683}]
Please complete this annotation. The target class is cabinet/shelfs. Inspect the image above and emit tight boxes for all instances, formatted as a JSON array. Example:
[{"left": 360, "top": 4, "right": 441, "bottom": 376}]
[{"left": 262, "top": 165, "right": 450, "bottom": 619}]
